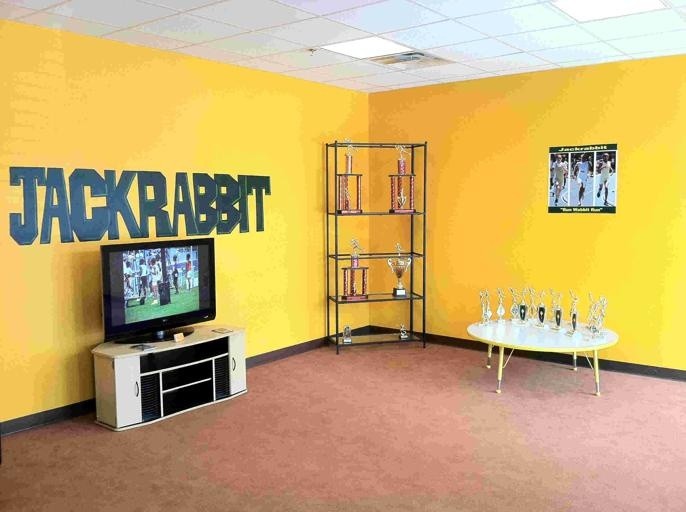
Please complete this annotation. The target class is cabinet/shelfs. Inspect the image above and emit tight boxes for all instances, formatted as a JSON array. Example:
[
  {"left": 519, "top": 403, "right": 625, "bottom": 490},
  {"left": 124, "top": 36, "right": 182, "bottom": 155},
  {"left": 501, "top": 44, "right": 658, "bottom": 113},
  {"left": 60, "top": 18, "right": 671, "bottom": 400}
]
[
  {"left": 325, "top": 140, "right": 429, "bottom": 355},
  {"left": 91, "top": 325, "right": 249, "bottom": 432}
]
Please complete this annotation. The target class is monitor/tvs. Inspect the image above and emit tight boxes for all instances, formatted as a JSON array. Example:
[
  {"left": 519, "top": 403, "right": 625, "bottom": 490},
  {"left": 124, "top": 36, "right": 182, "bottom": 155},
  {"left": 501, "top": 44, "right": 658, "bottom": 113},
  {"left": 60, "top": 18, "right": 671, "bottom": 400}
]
[{"left": 100, "top": 237, "right": 216, "bottom": 344}]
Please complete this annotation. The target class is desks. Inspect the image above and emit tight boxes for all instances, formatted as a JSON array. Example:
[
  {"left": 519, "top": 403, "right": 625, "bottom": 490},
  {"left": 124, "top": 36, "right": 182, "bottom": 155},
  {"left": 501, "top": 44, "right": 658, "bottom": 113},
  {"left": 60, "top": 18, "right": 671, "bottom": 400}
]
[{"left": 468, "top": 319, "right": 620, "bottom": 397}]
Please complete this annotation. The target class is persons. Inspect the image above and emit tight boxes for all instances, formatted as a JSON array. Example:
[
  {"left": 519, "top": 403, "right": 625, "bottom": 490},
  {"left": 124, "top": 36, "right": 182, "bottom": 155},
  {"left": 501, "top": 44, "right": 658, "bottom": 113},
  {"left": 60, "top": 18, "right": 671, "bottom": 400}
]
[
  {"left": 550, "top": 152, "right": 616, "bottom": 208},
  {"left": 122, "top": 244, "right": 201, "bottom": 309}
]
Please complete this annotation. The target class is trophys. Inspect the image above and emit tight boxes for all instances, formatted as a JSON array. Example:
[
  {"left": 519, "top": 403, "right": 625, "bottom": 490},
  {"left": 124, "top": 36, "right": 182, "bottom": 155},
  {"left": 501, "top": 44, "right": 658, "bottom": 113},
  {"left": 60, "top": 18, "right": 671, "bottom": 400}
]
[
  {"left": 387, "top": 241, "right": 414, "bottom": 297},
  {"left": 478, "top": 285, "right": 608, "bottom": 339}
]
